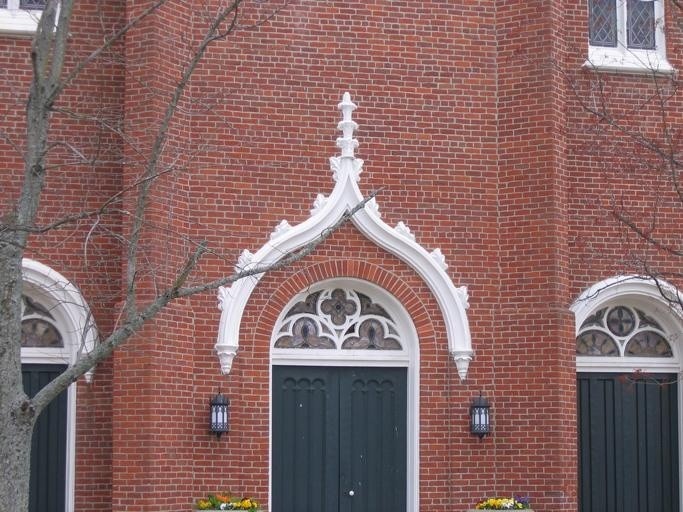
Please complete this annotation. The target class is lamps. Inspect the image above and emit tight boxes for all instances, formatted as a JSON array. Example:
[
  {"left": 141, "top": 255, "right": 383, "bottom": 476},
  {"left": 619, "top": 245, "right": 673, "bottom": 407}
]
[
  {"left": 470, "top": 391, "right": 491, "bottom": 439},
  {"left": 210, "top": 388, "right": 230, "bottom": 439}
]
